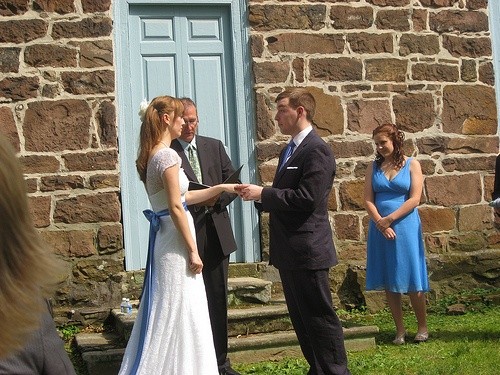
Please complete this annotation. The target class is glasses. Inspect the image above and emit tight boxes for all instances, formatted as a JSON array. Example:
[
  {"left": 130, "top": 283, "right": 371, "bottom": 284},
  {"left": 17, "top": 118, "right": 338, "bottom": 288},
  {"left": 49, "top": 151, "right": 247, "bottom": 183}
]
[{"left": 183, "top": 116, "right": 199, "bottom": 126}]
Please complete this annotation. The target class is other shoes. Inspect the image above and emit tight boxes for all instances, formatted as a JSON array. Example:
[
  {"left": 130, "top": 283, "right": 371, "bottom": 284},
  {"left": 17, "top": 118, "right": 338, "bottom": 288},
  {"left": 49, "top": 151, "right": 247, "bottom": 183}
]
[{"left": 221, "top": 367, "right": 242, "bottom": 375}]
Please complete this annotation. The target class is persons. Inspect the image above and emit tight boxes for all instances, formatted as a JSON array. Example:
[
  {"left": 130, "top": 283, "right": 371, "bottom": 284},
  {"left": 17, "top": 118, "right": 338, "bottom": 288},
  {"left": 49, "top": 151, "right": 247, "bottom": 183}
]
[
  {"left": 119, "top": 96, "right": 243, "bottom": 375},
  {"left": 492, "top": 153, "right": 500, "bottom": 232},
  {"left": 235, "top": 88, "right": 351, "bottom": 375},
  {"left": 172, "top": 97, "right": 242, "bottom": 375},
  {"left": 0, "top": 132, "right": 75, "bottom": 375},
  {"left": 366, "top": 124, "right": 429, "bottom": 344}
]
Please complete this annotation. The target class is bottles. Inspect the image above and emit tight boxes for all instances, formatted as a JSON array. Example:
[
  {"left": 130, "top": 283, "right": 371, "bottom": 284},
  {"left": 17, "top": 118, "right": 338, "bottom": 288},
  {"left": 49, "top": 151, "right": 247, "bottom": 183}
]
[
  {"left": 121, "top": 297, "right": 127, "bottom": 319},
  {"left": 124, "top": 298, "right": 133, "bottom": 319}
]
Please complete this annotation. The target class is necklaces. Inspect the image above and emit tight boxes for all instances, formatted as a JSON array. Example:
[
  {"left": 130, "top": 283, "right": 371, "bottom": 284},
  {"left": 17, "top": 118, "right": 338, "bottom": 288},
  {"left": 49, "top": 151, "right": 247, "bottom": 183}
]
[{"left": 159, "top": 140, "right": 168, "bottom": 148}]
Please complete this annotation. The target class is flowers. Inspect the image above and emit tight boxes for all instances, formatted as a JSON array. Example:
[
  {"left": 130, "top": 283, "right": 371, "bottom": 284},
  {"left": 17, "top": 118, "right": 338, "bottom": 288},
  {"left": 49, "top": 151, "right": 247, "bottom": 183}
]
[{"left": 137, "top": 100, "right": 150, "bottom": 119}]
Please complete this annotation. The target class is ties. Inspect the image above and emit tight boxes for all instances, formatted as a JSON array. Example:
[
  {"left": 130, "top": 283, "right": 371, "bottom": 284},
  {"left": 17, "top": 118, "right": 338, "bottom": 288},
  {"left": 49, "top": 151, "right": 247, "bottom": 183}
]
[
  {"left": 186, "top": 145, "right": 202, "bottom": 184},
  {"left": 278, "top": 141, "right": 296, "bottom": 172}
]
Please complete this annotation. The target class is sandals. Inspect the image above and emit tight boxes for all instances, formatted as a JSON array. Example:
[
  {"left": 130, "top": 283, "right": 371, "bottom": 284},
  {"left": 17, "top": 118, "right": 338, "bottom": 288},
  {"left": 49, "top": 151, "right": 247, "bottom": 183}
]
[
  {"left": 414, "top": 330, "right": 429, "bottom": 341},
  {"left": 394, "top": 333, "right": 406, "bottom": 344}
]
